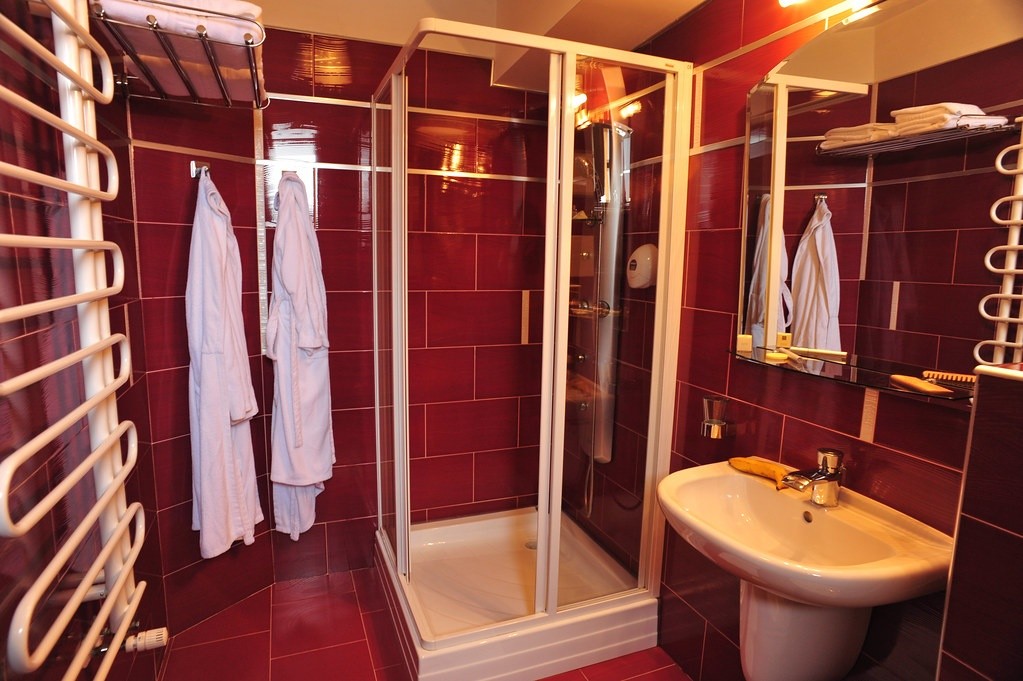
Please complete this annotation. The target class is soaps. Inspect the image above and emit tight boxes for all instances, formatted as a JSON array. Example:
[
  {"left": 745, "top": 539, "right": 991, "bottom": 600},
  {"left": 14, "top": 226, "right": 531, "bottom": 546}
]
[
  {"left": 735, "top": 333, "right": 753, "bottom": 353},
  {"left": 764, "top": 351, "right": 789, "bottom": 362},
  {"left": 774, "top": 332, "right": 794, "bottom": 348},
  {"left": 891, "top": 373, "right": 955, "bottom": 402}
]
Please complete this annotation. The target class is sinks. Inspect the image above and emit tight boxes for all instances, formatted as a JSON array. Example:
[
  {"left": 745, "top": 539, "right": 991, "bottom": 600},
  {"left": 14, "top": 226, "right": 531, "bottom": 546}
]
[{"left": 655, "top": 453, "right": 954, "bottom": 611}]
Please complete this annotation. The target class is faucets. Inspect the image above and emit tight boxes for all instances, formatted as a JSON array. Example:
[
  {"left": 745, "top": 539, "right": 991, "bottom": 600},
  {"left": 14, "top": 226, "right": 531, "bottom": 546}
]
[{"left": 778, "top": 447, "right": 847, "bottom": 510}]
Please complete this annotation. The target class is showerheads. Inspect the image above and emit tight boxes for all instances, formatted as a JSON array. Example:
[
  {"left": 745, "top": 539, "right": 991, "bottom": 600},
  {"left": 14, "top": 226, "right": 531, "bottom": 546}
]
[{"left": 574, "top": 154, "right": 607, "bottom": 223}]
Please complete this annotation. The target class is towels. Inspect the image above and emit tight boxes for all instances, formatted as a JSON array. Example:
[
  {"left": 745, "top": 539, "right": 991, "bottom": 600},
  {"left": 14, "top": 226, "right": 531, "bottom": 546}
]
[
  {"left": 816, "top": 102, "right": 1010, "bottom": 152},
  {"left": 90, "top": 0, "right": 268, "bottom": 103}
]
